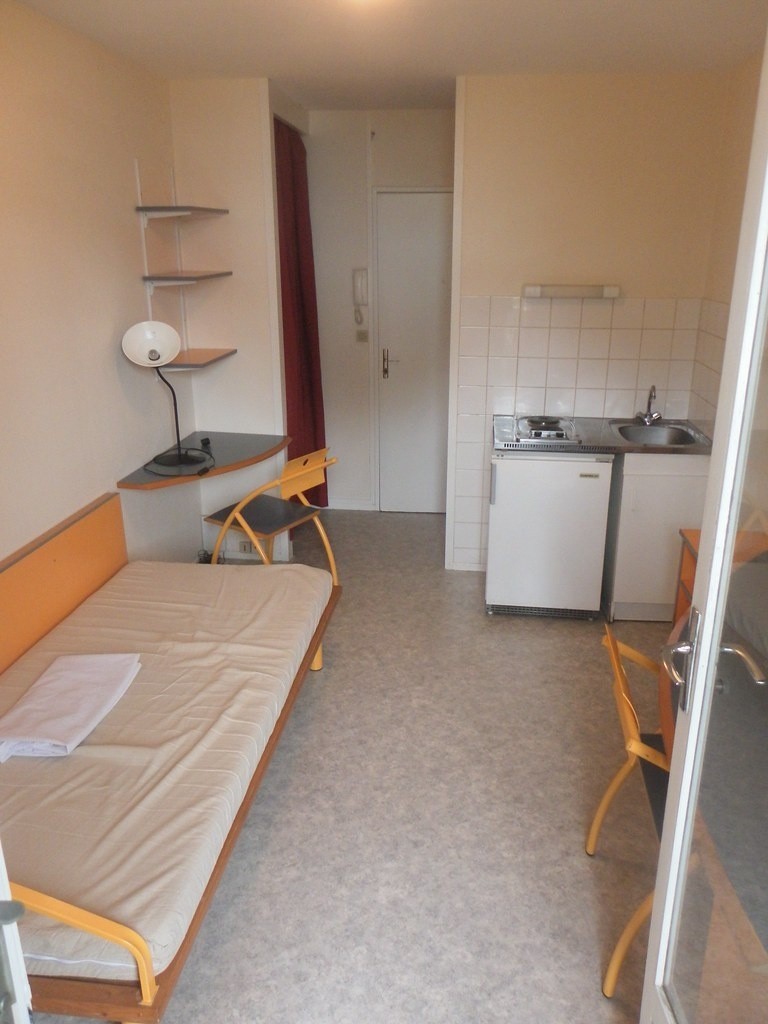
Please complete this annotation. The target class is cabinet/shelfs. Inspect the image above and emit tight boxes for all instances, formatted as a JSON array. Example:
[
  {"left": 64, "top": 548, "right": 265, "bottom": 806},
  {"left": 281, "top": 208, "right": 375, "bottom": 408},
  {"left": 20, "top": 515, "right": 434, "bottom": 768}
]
[
  {"left": 600, "top": 454, "right": 712, "bottom": 624},
  {"left": 674, "top": 527, "right": 768, "bottom": 623},
  {"left": 135, "top": 200, "right": 246, "bottom": 372}
]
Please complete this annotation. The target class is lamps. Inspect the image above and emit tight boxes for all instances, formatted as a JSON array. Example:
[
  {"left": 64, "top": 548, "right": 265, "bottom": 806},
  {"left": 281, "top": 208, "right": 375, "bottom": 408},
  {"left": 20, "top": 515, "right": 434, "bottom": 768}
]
[{"left": 122, "top": 321, "right": 216, "bottom": 477}]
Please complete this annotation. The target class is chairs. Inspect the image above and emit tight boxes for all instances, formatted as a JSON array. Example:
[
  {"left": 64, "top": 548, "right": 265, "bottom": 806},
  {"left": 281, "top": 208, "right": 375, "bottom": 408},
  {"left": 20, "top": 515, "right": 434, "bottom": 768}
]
[
  {"left": 204, "top": 448, "right": 357, "bottom": 670},
  {"left": 587, "top": 604, "right": 766, "bottom": 1021}
]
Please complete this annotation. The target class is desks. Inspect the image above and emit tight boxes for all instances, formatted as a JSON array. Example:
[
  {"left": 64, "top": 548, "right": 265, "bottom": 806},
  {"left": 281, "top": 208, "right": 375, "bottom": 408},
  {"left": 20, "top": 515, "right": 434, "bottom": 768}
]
[{"left": 121, "top": 431, "right": 292, "bottom": 564}]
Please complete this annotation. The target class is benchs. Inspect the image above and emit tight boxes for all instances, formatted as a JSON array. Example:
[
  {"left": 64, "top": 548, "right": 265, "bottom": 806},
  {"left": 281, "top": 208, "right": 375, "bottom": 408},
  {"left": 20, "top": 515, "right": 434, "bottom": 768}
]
[{"left": 0, "top": 490, "right": 345, "bottom": 1022}]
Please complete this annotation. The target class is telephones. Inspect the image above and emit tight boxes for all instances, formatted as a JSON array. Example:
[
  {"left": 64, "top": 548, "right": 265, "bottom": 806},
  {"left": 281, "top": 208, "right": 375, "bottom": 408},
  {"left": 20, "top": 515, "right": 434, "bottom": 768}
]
[{"left": 352, "top": 268, "right": 369, "bottom": 305}]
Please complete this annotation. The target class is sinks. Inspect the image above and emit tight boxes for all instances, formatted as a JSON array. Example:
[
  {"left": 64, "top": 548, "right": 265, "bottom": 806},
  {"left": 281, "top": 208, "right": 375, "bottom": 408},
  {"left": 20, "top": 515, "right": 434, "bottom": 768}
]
[{"left": 618, "top": 426, "right": 695, "bottom": 444}]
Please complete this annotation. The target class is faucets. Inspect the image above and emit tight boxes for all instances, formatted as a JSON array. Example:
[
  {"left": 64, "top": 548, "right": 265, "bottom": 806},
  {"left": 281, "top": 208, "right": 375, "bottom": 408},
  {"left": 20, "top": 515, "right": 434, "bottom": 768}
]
[{"left": 636, "top": 384, "right": 662, "bottom": 425}]
